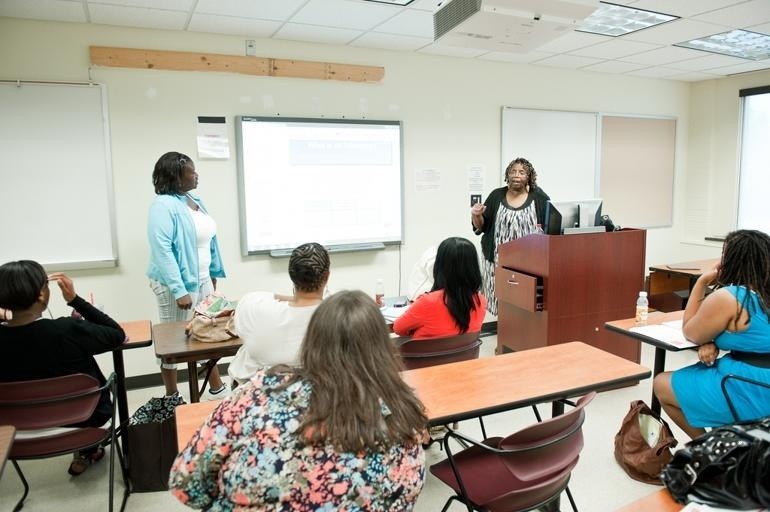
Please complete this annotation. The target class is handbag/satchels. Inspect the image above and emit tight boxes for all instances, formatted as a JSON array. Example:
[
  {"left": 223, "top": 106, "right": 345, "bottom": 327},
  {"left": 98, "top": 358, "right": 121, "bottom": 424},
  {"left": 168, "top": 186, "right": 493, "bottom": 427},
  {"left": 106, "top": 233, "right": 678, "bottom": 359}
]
[
  {"left": 184, "top": 290, "right": 239, "bottom": 342},
  {"left": 658, "top": 415, "right": 770, "bottom": 511},
  {"left": 613, "top": 399, "right": 677, "bottom": 486},
  {"left": 100, "top": 390, "right": 187, "bottom": 494}
]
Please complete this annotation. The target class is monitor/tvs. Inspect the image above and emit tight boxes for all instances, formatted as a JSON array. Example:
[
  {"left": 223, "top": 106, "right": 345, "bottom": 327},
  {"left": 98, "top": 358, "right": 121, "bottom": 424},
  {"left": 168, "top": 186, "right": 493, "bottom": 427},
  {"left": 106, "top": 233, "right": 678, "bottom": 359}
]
[{"left": 543, "top": 198, "right": 605, "bottom": 235}]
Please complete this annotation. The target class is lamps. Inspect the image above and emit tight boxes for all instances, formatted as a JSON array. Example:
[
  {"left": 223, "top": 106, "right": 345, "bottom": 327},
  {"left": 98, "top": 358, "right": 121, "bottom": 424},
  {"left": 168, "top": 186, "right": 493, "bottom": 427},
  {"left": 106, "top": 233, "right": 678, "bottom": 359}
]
[
  {"left": 672, "top": 29, "right": 770, "bottom": 61},
  {"left": 574, "top": 1, "right": 680, "bottom": 38}
]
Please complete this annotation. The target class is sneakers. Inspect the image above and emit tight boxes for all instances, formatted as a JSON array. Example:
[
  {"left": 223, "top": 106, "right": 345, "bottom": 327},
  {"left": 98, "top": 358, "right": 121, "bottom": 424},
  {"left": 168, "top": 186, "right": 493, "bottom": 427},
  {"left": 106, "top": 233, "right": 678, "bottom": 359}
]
[{"left": 208, "top": 382, "right": 231, "bottom": 400}]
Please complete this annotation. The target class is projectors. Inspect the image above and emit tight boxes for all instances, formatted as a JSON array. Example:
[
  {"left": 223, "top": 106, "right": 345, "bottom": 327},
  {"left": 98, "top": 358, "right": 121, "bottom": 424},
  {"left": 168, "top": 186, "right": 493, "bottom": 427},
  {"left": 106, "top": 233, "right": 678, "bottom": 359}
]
[{"left": 432, "top": 1, "right": 600, "bottom": 60}]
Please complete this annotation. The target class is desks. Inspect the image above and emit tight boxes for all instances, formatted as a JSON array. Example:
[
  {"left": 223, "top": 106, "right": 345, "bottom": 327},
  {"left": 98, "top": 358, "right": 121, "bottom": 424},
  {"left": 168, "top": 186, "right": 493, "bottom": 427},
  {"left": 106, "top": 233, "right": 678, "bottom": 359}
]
[
  {"left": 0, "top": 425, "right": 22, "bottom": 476},
  {"left": 604, "top": 308, "right": 748, "bottom": 421},
  {"left": 648, "top": 258, "right": 728, "bottom": 310},
  {"left": 494, "top": 229, "right": 649, "bottom": 393},
  {"left": 613, "top": 485, "right": 683, "bottom": 512},
  {"left": 112, "top": 320, "right": 152, "bottom": 476},
  {"left": 90, "top": 319, "right": 154, "bottom": 456},
  {"left": 152, "top": 295, "right": 498, "bottom": 405},
  {"left": 173, "top": 399, "right": 229, "bottom": 454},
  {"left": 175, "top": 341, "right": 652, "bottom": 460},
  {"left": 644, "top": 362, "right": 644, "bottom": 366},
  {"left": 152, "top": 326, "right": 248, "bottom": 395}
]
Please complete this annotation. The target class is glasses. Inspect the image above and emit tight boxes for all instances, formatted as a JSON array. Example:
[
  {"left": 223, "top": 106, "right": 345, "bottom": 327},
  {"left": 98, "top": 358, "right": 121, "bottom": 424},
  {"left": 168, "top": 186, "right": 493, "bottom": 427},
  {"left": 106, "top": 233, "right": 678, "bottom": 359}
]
[
  {"left": 179, "top": 154, "right": 189, "bottom": 165},
  {"left": 508, "top": 171, "right": 525, "bottom": 177}
]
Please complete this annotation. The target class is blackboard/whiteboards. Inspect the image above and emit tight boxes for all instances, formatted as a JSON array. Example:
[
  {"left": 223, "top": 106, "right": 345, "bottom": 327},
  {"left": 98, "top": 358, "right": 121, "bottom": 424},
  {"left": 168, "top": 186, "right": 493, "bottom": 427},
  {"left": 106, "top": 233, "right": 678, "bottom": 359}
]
[
  {"left": 599, "top": 112, "right": 678, "bottom": 229},
  {"left": 235, "top": 115, "right": 404, "bottom": 258},
  {"left": 0, "top": 77, "right": 120, "bottom": 274},
  {"left": 500, "top": 105, "right": 598, "bottom": 201}
]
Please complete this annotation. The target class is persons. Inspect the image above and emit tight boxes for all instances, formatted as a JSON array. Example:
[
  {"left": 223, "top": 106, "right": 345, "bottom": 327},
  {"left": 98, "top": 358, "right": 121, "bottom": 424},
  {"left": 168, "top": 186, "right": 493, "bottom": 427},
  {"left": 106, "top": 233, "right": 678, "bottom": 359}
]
[
  {"left": 143, "top": 150, "right": 231, "bottom": 402},
  {"left": 168, "top": 291, "right": 427, "bottom": 512},
  {"left": 653, "top": 228, "right": 770, "bottom": 438},
  {"left": 393, "top": 237, "right": 486, "bottom": 341},
  {"left": 470, "top": 158, "right": 550, "bottom": 314},
  {"left": 0, "top": 259, "right": 126, "bottom": 477},
  {"left": 226, "top": 243, "right": 329, "bottom": 383}
]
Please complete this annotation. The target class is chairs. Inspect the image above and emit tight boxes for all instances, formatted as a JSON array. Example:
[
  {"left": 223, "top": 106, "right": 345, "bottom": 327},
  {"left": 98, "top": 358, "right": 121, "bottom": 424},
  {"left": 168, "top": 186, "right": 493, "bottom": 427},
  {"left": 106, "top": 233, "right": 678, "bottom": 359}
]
[
  {"left": 429, "top": 390, "right": 599, "bottom": 512},
  {"left": 2, "top": 373, "right": 131, "bottom": 512},
  {"left": 717, "top": 374, "right": 765, "bottom": 419},
  {"left": 393, "top": 333, "right": 487, "bottom": 442}
]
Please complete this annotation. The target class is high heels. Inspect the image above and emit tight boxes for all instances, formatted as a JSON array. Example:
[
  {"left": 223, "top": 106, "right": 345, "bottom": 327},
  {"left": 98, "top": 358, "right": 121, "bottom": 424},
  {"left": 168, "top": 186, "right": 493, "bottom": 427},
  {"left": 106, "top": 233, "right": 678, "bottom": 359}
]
[
  {"left": 87, "top": 446, "right": 106, "bottom": 465},
  {"left": 68, "top": 454, "right": 87, "bottom": 477}
]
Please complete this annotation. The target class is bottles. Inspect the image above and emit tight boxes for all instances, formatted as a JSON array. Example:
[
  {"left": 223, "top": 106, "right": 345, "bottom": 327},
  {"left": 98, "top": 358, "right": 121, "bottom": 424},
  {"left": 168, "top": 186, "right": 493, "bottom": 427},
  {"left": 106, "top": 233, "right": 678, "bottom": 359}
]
[
  {"left": 636, "top": 291, "right": 648, "bottom": 323},
  {"left": 376, "top": 280, "right": 385, "bottom": 308},
  {"left": 534, "top": 224, "right": 544, "bottom": 235}
]
